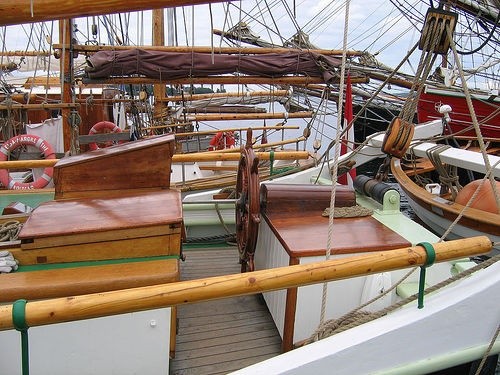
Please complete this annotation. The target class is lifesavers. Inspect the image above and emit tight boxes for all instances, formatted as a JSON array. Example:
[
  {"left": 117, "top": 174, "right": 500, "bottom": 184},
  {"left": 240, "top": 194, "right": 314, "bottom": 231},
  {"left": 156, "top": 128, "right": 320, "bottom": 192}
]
[
  {"left": 208, "top": 132, "right": 223, "bottom": 151},
  {"left": 223, "top": 131, "right": 236, "bottom": 149},
  {"left": 88, "top": 121, "right": 123, "bottom": 151},
  {"left": 0, "top": 134, "right": 55, "bottom": 191}
]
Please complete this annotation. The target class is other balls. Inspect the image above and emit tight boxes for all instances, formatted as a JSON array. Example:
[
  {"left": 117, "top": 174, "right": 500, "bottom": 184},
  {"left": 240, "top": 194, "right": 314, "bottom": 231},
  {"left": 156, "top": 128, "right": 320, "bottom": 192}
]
[{"left": 453, "top": 179, "right": 500, "bottom": 215}]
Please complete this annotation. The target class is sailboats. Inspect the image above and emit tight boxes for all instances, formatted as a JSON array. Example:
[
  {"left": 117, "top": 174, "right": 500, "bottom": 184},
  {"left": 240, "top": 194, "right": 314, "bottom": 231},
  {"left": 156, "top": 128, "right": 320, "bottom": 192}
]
[{"left": 2, "top": 0, "right": 499, "bottom": 374}]
